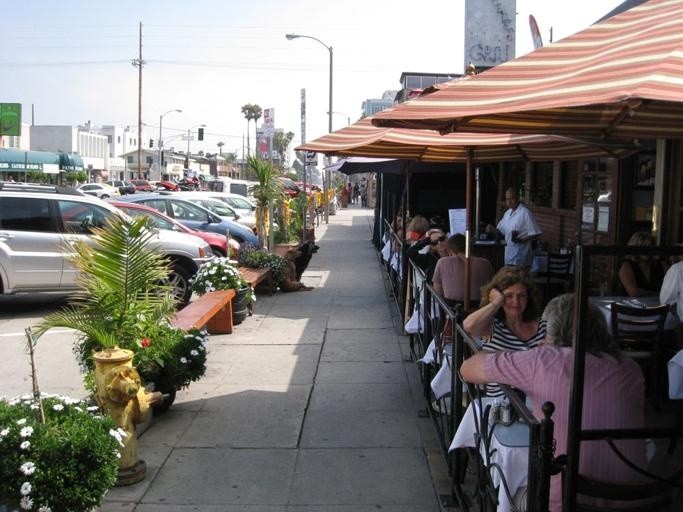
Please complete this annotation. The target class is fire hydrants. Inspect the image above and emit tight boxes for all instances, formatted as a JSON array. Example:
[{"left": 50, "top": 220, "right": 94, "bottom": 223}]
[{"left": 84, "top": 347, "right": 170, "bottom": 487}]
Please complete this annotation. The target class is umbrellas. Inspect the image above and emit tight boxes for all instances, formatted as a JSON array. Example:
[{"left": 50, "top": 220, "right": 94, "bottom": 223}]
[
  {"left": 364, "top": 0, "right": 682, "bottom": 140},
  {"left": 318, "top": 156, "right": 481, "bottom": 218},
  {"left": 295, "top": 69, "right": 633, "bottom": 254}
]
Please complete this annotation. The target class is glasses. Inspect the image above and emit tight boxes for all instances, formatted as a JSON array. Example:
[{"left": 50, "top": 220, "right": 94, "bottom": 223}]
[{"left": 431, "top": 235, "right": 445, "bottom": 245}]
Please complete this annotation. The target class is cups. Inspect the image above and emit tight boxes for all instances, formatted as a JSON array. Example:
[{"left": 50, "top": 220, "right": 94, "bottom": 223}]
[{"left": 511, "top": 229, "right": 518, "bottom": 240}]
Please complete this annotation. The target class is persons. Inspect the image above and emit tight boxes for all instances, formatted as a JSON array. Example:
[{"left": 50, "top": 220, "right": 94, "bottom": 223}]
[
  {"left": 346, "top": 182, "right": 368, "bottom": 207},
  {"left": 482, "top": 186, "right": 541, "bottom": 267},
  {"left": 405, "top": 228, "right": 448, "bottom": 298},
  {"left": 458, "top": 293, "right": 647, "bottom": 511},
  {"left": 610, "top": 230, "right": 662, "bottom": 296},
  {"left": 431, "top": 233, "right": 491, "bottom": 312},
  {"left": 461, "top": 265, "right": 548, "bottom": 398},
  {"left": 406, "top": 215, "right": 429, "bottom": 240}
]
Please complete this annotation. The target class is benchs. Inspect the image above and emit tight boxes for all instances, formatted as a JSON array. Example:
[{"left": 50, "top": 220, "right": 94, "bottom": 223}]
[
  {"left": 161, "top": 291, "right": 234, "bottom": 352},
  {"left": 230, "top": 267, "right": 273, "bottom": 311}
]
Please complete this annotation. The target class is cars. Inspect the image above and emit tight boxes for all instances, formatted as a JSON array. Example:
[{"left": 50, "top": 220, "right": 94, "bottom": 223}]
[
  {"left": 60, "top": 194, "right": 258, "bottom": 254},
  {"left": 159, "top": 174, "right": 324, "bottom": 239},
  {"left": 41, "top": 201, "right": 240, "bottom": 263},
  {"left": 76, "top": 176, "right": 199, "bottom": 200}
]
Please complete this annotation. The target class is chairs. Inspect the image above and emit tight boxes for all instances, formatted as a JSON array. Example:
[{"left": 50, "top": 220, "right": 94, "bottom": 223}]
[
  {"left": 545, "top": 253, "right": 571, "bottom": 291},
  {"left": 607, "top": 304, "right": 667, "bottom": 390},
  {"left": 564, "top": 425, "right": 680, "bottom": 512}
]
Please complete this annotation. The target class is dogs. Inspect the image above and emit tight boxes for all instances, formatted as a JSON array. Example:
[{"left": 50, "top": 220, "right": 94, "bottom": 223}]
[
  {"left": 280, "top": 245, "right": 315, "bottom": 292},
  {"left": 294, "top": 240, "right": 320, "bottom": 283}
]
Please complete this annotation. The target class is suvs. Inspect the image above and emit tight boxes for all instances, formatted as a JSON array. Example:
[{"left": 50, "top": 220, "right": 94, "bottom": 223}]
[{"left": 0, "top": 180, "right": 214, "bottom": 312}]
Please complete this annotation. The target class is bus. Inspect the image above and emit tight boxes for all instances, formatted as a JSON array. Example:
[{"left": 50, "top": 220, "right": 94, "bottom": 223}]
[{"left": 0, "top": 148, "right": 84, "bottom": 188}]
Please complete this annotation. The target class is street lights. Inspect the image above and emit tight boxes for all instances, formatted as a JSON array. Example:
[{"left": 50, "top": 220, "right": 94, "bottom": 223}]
[
  {"left": 186, "top": 124, "right": 206, "bottom": 177},
  {"left": 234, "top": 145, "right": 252, "bottom": 179},
  {"left": 326, "top": 110, "right": 352, "bottom": 126},
  {"left": 285, "top": 32, "right": 334, "bottom": 226},
  {"left": 156, "top": 109, "right": 182, "bottom": 181}
]
